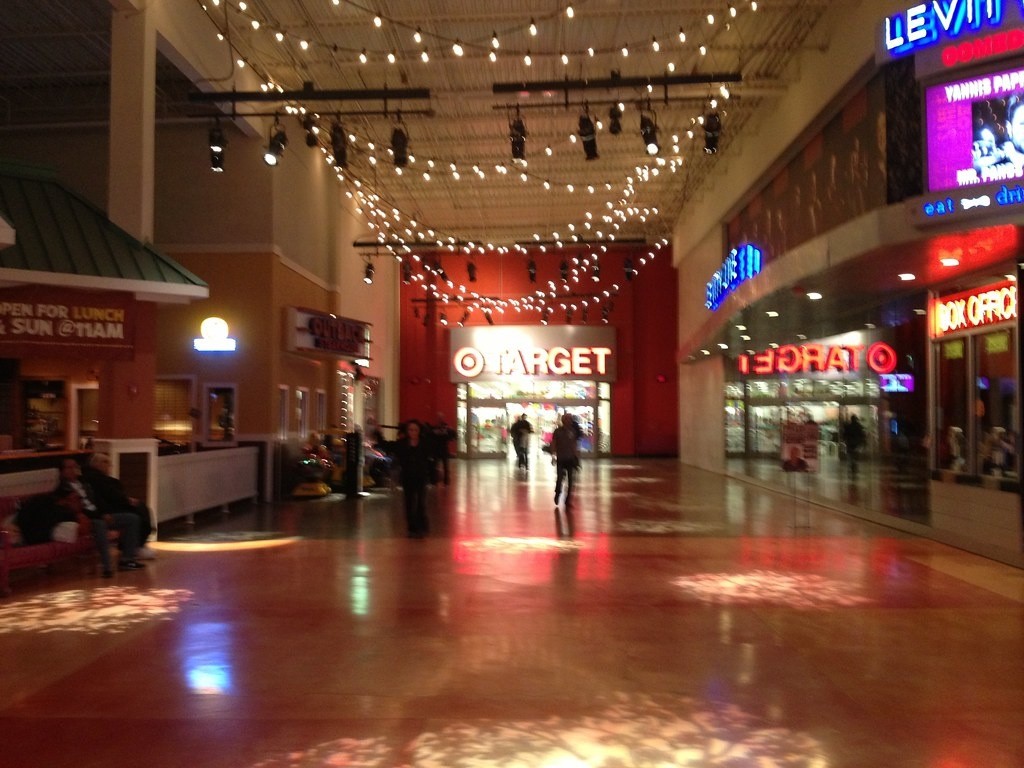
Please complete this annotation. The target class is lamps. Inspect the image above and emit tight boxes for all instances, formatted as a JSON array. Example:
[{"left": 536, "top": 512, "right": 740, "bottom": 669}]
[
  {"left": 575, "top": 102, "right": 600, "bottom": 163},
  {"left": 700, "top": 99, "right": 722, "bottom": 155},
  {"left": 592, "top": 261, "right": 601, "bottom": 282},
  {"left": 263, "top": 139, "right": 283, "bottom": 167},
  {"left": 390, "top": 110, "right": 414, "bottom": 169},
  {"left": 624, "top": 258, "right": 633, "bottom": 281},
  {"left": 609, "top": 102, "right": 623, "bottom": 135},
  {"left": 210, "top": 151, "right": 227, "bottom": 175},
  {"left": 640, "top": 100, "right": 663, "bottom": 157},
  {"left": 272, "top": 112, "right": 289, "bottom": 150},
  {"left": 508, "top": 106, "right": 527, "bottom": 163},
  {"left": 209, "top": 115, "right": 228, "bottom": 153},
  {"left": 401, "top": 254, "right": 413, "bottom": 285},
  {"left": 363, "top": 254, "right": 376, "bottom": 285},
  {"left": 418, "top": 253, "right": 589, "bottom": 326},
  {"left": 601, "top": 307, "right": 608, "bottom": 324},
  {"left": 329, "top": 123, "right": 349, "bottom": 171}
]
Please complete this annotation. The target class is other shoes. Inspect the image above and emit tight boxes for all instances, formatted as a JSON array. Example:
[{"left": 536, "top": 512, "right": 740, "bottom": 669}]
[
  {"left": 103, "top": 569, "right": 113, "bottom": 578},
  {"left": 118, "top": 561, "right": 145, "bottom": 570},
  {"left": 554, "top": 495, "right": 559, "bottom": 504},
  {"left": 565, "top": 496, "right": 573, "bottom": 503}
]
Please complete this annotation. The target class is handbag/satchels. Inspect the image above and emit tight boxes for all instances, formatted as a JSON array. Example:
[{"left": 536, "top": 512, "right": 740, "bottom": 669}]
[{"left": 560, "top": 455, "right": 580, "bottom": 470}]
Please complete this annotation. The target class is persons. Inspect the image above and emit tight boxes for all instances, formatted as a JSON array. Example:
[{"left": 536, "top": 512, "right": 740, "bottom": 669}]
[
  {"left": 550, "top": 414, "right": 582, "bottom": 509},
  {"left": 516, "top": 413, "right": 534, "bottom": 469},
  {"left": 87, "top": 453, "right": 152, "bottom": 557},
  {"left": 510, "top": 416, "right": 522, "bottom": 450},
  {"left": 427, "top": 412, "right": 458, "bottom": 485},
  {"left": 842, "top": 416, "right": 867, "bottom": 476},
  {"left": 370, "top": 416, "right": 431, "bottom": 538},
  {"left": 53, "top": 458, "right": 146, "bottom": 579},
  {"left": 972, "top": 99, "right": 1024, "bottom": 171},
  {"left": 302, "top": 424, "right": 395, "bottom": 491}
]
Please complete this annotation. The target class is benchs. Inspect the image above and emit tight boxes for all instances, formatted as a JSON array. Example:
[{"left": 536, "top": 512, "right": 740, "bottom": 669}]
[{"left": 0, "top": 490, "right": 122, "bottom": 596}]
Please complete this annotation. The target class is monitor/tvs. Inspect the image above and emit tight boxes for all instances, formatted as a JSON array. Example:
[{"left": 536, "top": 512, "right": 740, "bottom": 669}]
[{"left": 923, "top": 57, "right": 1024, "bottom": 192}]
